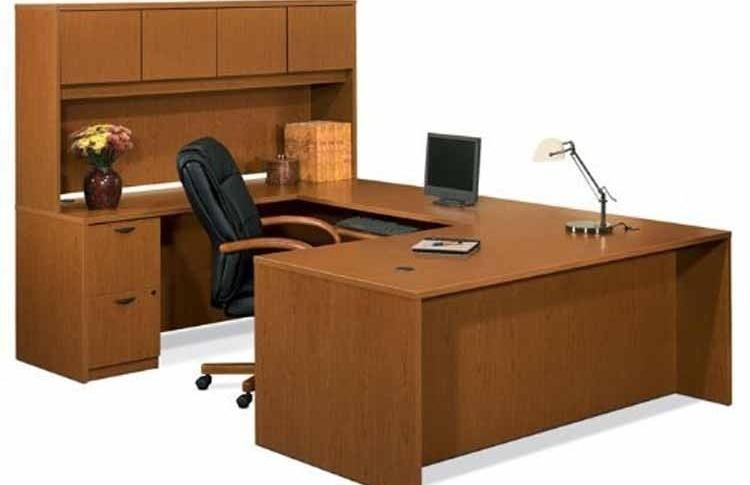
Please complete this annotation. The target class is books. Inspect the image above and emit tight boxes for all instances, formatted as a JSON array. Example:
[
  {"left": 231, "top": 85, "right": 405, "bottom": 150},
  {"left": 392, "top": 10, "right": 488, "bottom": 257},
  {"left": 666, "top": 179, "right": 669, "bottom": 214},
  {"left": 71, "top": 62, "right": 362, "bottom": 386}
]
[{"left": 287, "top": 118, "right": 350, "bottom": 185}]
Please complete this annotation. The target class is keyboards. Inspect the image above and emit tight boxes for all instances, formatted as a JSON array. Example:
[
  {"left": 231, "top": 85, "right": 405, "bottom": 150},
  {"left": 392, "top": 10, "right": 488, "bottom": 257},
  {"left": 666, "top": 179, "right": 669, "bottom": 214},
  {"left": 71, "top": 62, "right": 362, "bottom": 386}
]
[{"left": 335, "top": 216, "right": 421, "bottom": 243}]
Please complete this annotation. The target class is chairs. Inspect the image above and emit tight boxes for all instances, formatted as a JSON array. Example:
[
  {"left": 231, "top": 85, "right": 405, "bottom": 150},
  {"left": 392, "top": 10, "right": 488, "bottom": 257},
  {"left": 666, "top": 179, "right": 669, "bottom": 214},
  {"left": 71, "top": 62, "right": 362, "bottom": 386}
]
[{"left": 174, "top": 135, "right": 342, "bottom": 408}]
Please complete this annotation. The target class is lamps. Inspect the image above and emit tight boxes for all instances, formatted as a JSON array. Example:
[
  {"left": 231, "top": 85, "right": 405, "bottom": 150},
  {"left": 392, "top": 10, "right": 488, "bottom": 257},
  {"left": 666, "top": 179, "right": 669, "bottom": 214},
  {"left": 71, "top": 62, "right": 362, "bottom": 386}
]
[{"left": 532, "top": 137, "right": 641, "bottom": 236}]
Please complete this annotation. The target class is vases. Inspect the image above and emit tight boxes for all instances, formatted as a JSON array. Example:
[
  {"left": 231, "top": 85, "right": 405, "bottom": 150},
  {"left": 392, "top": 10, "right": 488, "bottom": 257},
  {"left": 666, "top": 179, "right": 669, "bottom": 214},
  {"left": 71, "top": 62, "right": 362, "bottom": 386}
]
[{"left": 84, "top": 162, "right": 121, "bottom": 210}]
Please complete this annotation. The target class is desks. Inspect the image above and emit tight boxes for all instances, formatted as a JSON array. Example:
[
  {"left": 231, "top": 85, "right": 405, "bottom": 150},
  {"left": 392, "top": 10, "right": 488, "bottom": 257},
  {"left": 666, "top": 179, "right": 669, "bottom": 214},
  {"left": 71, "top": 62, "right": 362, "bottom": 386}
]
[{"left": 254, "top": 179, "right": 731, "bottom": 482}]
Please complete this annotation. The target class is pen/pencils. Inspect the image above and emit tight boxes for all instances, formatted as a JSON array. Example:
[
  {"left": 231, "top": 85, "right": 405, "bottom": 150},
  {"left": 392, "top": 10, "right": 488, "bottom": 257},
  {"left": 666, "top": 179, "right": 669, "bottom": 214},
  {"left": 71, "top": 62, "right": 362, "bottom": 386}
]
[{"left": 432, "top": 240, "right": 461, "bottom": 246}]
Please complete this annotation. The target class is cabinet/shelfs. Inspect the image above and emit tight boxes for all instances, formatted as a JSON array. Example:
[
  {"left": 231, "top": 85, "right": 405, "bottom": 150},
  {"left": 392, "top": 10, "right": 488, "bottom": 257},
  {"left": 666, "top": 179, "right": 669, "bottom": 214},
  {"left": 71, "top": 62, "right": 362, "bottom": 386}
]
[{"left": 15, "top": 0, "right": 357, "bottom": 383}]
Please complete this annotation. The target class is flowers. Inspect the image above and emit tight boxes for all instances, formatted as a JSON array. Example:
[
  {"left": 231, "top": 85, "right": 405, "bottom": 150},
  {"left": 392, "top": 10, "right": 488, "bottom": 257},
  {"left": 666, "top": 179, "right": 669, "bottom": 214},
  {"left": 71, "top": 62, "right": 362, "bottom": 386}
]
[{"left": 67, "top": 123, "right": 133, "bottom": 167}]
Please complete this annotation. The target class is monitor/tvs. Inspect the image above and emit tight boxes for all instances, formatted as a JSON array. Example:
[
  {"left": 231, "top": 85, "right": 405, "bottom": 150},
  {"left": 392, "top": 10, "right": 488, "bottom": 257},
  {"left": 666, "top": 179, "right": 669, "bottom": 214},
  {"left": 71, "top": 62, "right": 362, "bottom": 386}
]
[{"left": 423, "top": 132, "right": 482, "bottom": 208}]
